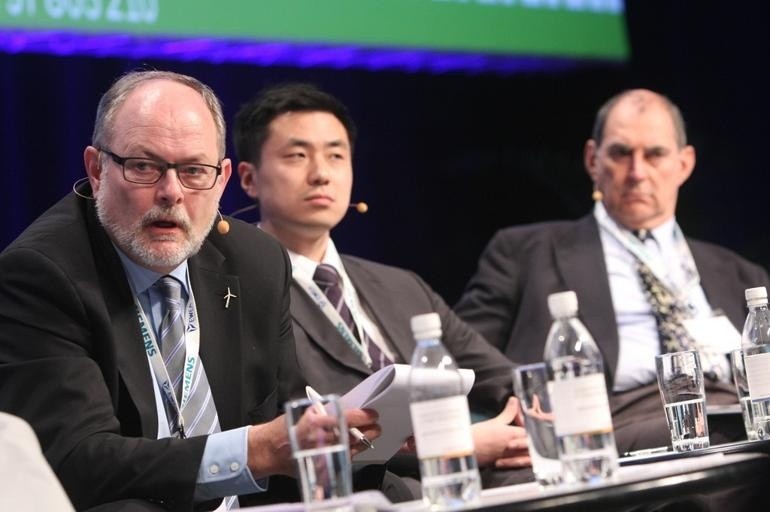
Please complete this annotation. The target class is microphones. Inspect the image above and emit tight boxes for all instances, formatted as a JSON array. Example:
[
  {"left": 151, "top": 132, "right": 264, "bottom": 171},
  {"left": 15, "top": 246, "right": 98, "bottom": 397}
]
[
  {"left": 349, "top": 201, "right": 369, "bottom": 214},
  {"left": 591, "top": 183, "right": 603, "bottom": 201},
  {"left": 217, "top": 209, "right": 230, "bottom": 235}
]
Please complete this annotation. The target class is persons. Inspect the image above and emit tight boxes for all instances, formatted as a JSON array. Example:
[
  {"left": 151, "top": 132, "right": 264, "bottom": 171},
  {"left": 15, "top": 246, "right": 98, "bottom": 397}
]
[
  {"left": 0, "top": 69, "right": 383, "bottom": 511},
  {"left": 233, "top": 82, "right": 542, "bottom": 508},
  {"left": 440, "top": 89, "right": 770, "bottom": 460}
]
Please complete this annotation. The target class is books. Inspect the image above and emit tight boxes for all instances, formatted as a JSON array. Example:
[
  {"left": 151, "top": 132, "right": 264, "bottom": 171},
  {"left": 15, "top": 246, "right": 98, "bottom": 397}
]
[{"left": 316, "top": 363, "right": 476, "bottom": 464}]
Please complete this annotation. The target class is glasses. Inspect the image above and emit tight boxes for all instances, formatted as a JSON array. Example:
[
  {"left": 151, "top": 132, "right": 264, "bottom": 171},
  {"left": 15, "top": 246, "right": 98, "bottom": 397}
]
[{"left": 98, "top": 145, "right": 222, "bottom": 190}]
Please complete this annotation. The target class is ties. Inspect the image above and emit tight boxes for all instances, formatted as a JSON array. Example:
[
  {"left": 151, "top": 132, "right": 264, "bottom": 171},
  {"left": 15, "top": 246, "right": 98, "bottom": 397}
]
[
  {"left": 314, "top": 264, "right": 393, "bottom": 371},
  {"left": 157, "top": 277, "right": 240, "bottom": 512},
  {"left": 631, "top": 230, "right": 721, "bottom": 381}
]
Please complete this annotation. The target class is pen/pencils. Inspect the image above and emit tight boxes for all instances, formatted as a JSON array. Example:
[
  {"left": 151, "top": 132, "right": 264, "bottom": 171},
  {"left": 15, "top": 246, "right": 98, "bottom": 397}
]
[
  {"left": 305, "top": 386, "right": 374, "bottom": 449},
  {"left": 624, "top": 446, "right": 673, "bottom": 456}
]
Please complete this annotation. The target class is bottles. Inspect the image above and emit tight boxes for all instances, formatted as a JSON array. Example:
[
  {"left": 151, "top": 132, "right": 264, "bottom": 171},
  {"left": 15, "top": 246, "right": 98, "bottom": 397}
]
[
  {"left": 404, "top": 309, "right": 485, "bottom": 506},
  {"left": 543, "top": 291, "right": 619, "bottom": 483},
  {"left": 741, "top": 287, "right": 770, "bottom": 441}
]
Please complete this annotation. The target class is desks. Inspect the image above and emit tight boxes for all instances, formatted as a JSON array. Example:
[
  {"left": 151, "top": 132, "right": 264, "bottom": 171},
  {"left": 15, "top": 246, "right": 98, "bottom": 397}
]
[{"left": 359, "top": 438, "right": 770, "bottom": 510}]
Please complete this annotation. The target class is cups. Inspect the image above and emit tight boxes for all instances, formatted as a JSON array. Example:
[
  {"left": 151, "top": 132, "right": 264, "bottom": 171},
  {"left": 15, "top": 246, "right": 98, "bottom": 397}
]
[
  {"left": 654, "top": 351, "right": 710, "bottom": 454},
  {"left": 729, "top": 345, "right": 767, "bottom": 437},
  {"left": 285, "top": 394, "right": 353, "bottom": 498},
  {"left": 507, "top": 361, "right": 563, "bottom": 482}
]
